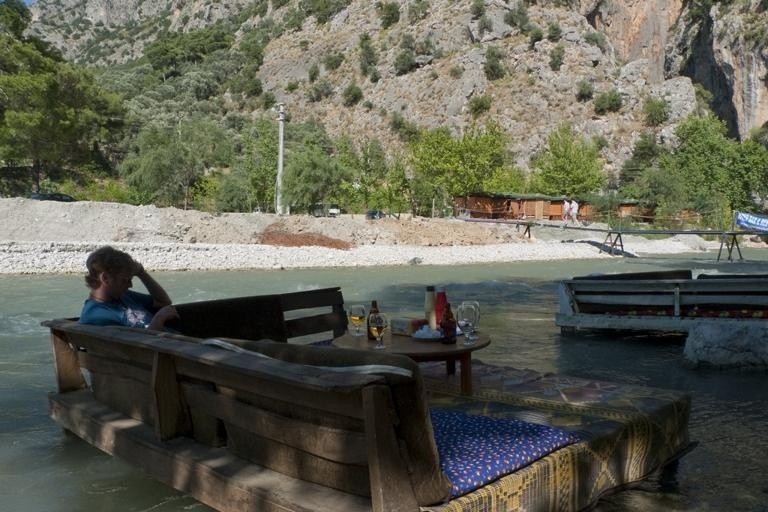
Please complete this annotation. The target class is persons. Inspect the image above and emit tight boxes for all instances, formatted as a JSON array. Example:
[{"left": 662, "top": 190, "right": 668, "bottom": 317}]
[
  {"left": 561, "top": 197, "right": 581, "bottom": 228},
  {"left": 78, "top": 245, "right": 187, "bottom": 334}
]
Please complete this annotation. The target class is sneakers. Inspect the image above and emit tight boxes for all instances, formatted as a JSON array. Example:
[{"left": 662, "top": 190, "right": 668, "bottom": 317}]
[{"left": 563, "top": 222, "right": 581, "bottom": 226}]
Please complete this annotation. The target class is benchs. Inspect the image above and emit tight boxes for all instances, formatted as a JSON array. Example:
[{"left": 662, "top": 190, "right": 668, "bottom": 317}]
[{"left": 40, "top": 285, "right": 698, "bottom": 508}]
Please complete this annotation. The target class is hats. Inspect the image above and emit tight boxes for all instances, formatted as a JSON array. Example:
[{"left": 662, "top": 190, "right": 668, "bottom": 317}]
[{"left": 86, "top": 245, "right": 121, "bottom": 276}]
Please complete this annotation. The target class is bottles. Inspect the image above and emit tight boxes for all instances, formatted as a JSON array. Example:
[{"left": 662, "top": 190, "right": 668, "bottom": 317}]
[
  {"left": 435, "top": 286, "right": 448, "bottom": 330},
  {"left": 439, "top": 303, "right": 457, "bottom": 345},
  {"left": 424, "top": 286, "right": 437, "bottom": 330},
  {"left": 367, "top": 300, "right": 380, "bottom": 341}
]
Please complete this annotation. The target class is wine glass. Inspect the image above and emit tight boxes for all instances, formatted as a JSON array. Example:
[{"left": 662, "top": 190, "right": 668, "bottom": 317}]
[
  {"left": 457, "top": 304, "right": 477, "bottom": 346},
  {"left": 369, "top": 312, "right": 388, "bottom": 350},
  {"left": 463, "top": 300, "right": 480, "bottom": 341},
  {"left": 350, "top": 304, "right": 366, "bottom": 337}
]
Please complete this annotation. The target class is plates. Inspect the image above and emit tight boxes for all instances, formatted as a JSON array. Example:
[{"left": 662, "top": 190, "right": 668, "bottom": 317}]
[{"left": 411, "top": 329, "right": 444, "bottom": 342}]
